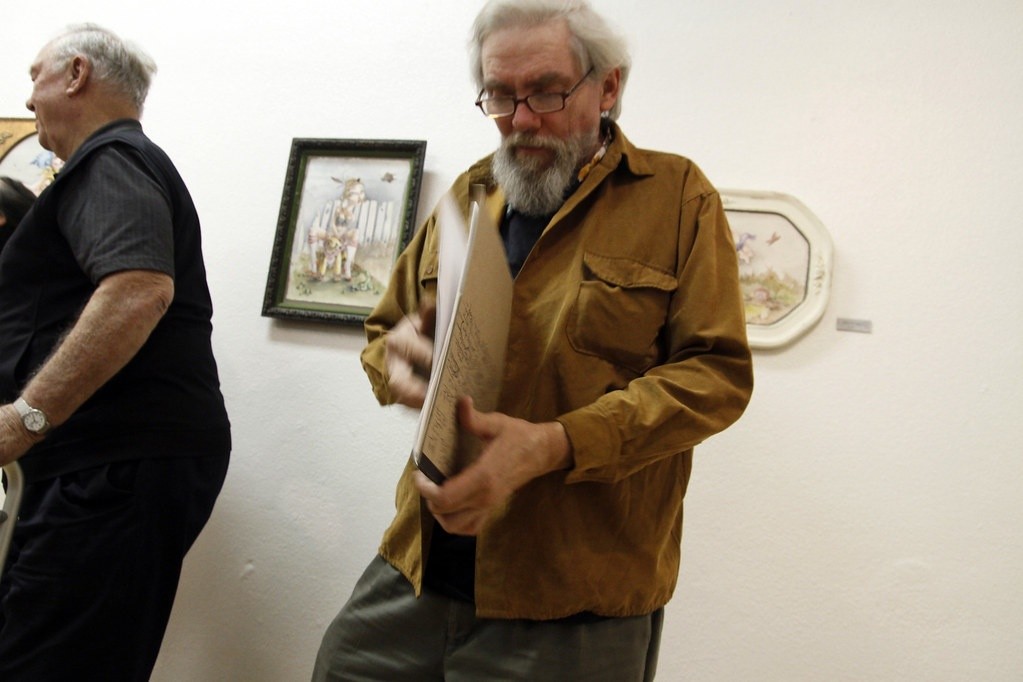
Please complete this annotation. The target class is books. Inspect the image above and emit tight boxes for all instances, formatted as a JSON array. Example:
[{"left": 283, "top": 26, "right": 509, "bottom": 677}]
[{"left": 410, "top": 181, "right": 515, "bottom": 487}]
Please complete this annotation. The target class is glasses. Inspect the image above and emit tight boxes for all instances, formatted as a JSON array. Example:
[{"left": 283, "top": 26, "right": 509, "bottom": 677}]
[{"left": 473, "top": 66, "right": 595, "bottom": 120}]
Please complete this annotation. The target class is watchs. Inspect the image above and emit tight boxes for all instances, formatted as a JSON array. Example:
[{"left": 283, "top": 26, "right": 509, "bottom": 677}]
[{"left": 12, "top": 399, "right": 55, "bottom": 438}]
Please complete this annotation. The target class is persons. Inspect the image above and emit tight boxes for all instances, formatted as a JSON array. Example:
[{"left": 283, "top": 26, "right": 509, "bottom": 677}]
[
  {"left": 312, "top": 0, "right": 757, "bottom": 681},
  {"left": 0, "top": 26, "right": 235, "bottom": 682}
]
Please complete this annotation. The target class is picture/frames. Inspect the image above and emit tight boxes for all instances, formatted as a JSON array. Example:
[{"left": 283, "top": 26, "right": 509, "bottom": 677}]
[
  {"left": 262, "top": 138, "right": 427, "bottom": 327},
  {"left": 716, "top": 190, "right": 829, "bottom": 349},
  {"left": 0, "top": 118, "right": 65, "bottom": 198}
]
